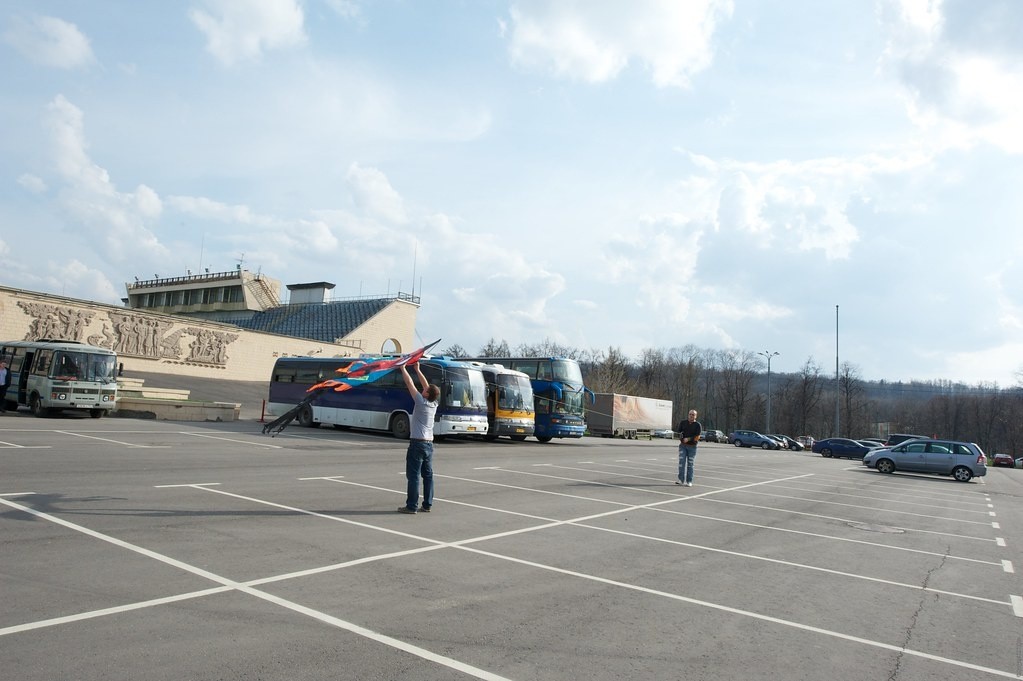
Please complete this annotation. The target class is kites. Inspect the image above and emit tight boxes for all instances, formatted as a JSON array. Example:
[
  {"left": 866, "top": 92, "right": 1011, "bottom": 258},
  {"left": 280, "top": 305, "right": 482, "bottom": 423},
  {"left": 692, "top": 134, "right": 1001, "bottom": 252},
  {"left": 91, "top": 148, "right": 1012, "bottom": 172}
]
[{"left": 262, "top": 338, "right": 442, "bottom": 437}]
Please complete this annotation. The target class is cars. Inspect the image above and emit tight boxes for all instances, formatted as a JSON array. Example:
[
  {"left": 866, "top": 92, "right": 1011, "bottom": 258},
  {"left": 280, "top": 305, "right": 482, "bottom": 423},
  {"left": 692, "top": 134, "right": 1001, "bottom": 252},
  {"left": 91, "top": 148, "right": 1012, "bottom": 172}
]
[
  {"left": 992, "top": 454, "right": 1014, "bottom": 468},
  {"left": 796, "top": 436, "right": 816, "bottom": 448},
  {"left": 699, "top": 430, "right": 706, "bottom": 440},
  {"left": 655, "top": 430, "right": 674, "bottom": 439},
  {"left": 856, "top": 438, "right": 887, "bottom": 448},
  {"left": 762, "top": 434, "right": 786, "bottom": 450},
  {"left": 776, "top": 435, "right": 805, "bottom": 451},
  {"left": 864, "top": 439, "right": 987, "bottom": 482},
  {"left": 1015, "top": 457, "right": 1023, "bottom": 469},
  {"left": 729, "top": 430, "right": 776, "bottom": 449},
  {"left": 812, "top": 439, "right": 877, "bottom": 459}
]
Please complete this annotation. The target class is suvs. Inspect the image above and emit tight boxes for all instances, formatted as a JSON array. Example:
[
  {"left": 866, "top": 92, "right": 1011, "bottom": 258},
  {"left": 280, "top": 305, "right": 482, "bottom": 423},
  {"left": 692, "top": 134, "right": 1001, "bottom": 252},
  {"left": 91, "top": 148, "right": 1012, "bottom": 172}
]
[{"left": 706, "top": 430, "right": 729, "bottom": 444}]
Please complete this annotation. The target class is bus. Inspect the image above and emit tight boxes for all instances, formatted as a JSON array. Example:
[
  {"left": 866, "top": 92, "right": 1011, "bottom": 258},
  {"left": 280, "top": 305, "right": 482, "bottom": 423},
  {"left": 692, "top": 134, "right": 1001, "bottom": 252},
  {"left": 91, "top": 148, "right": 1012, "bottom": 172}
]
[
  {"left": 267, "top": 355, "right": 490, "bottom": 440},
  {"left": 471, "top": 362, "right": 537, "bottom": 442},
  {"left": 0, "top": 338, "right": 124, "bottom": 417},
  {"left": 451, "top": 358, "right": 596, "bottom": 444}
]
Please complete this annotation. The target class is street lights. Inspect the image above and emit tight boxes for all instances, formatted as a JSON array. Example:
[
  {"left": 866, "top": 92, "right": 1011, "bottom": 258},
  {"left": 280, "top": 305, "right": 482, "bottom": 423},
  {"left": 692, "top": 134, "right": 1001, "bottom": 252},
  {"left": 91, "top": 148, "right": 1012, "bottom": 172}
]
[
  {"left": 836, "top": 305, "right": 839, "bottom": 436},
  {"left": 758, "top": 351, "right": 780, "bottom": 433}
]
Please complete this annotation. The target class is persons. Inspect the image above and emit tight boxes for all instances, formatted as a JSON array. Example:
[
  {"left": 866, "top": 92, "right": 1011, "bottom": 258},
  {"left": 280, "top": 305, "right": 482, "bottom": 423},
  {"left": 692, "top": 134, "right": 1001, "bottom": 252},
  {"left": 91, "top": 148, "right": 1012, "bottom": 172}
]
[
  {"left": 0, "top": 362, "right": 12, "bottom": 413},
  {"left": 398, "top": 361, "right": 440, "bottom": 514},
  {"left": 675, "top": 409, "right": 702, "bottom": 487}
]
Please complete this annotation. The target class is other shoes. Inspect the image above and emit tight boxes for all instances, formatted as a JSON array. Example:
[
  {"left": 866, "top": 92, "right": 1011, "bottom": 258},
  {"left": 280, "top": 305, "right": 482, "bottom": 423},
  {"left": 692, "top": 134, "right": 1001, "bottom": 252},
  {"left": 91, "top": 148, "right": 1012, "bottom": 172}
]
[
  {"left": 676, "top": 480, "right": 684, "bottom": 484},
  {"left": 421, "top": 505, "right": 431, "bottom": 512},
  {"left": 687, "top": 481, "right": 692, "bottom": 486},
  {"left": 398, "top": 505, "right": 417, "bottom": 514}
]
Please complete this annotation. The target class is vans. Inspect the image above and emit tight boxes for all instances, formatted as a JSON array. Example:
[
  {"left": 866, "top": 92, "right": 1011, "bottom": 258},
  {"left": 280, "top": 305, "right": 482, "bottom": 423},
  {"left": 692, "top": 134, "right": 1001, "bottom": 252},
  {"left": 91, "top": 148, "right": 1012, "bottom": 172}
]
[{"left": 885, "top": 434, "right": 930, "bottom": 445}]
